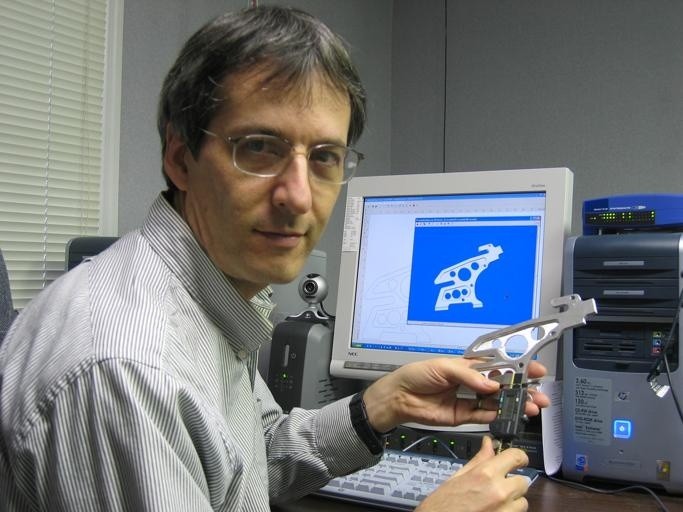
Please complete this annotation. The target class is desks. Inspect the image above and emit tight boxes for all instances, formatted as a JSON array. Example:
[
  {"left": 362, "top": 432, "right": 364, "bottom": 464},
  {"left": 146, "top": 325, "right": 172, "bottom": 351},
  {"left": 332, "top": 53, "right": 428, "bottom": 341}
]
[{"left": 268, "top": 412, "right": 683, "bottom": 512}]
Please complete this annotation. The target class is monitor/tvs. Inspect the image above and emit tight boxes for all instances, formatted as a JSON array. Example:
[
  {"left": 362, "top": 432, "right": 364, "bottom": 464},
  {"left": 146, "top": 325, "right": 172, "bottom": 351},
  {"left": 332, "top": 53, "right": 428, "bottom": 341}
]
[{"left": 327, "top": 165, "right": 575, "bottom": 431}]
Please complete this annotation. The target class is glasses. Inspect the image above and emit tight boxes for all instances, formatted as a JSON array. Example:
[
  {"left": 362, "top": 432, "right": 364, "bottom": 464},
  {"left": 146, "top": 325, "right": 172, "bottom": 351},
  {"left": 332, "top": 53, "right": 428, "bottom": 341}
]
[{"left": 190, "top": 124, "right": 365, "bottom": 186}]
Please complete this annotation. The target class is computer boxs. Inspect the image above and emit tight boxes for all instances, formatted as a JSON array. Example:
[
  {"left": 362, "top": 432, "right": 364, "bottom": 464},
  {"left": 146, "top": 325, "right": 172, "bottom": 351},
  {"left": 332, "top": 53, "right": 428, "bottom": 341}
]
[{"left": 561, "top": 233, "right": 682, "bottom": 495}]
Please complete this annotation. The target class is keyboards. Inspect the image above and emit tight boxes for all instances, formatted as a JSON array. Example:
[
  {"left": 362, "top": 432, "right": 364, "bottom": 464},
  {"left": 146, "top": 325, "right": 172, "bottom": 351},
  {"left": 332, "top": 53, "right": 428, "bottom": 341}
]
[{"left": 312, "top": 447, "right": 539, "bottom": 511}]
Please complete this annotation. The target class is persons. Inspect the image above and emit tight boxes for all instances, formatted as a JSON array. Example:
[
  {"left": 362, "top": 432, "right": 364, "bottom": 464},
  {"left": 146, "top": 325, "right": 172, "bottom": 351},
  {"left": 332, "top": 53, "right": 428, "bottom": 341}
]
[{"left": 1, "top": 4, "right": 549, "bottom": 512}]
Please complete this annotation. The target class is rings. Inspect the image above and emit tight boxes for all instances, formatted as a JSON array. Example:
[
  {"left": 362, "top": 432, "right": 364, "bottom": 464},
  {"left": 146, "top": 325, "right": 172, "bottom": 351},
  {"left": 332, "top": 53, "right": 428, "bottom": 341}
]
[{"left": 475, "top": 395, "right": 482, "bottom": 408}]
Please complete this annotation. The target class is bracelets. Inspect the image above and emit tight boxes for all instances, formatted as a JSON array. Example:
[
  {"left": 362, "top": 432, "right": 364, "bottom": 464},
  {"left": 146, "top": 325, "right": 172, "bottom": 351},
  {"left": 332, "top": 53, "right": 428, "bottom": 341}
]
[{"left": 348, "top": 385, "right": 399, "bottom": 457}]
